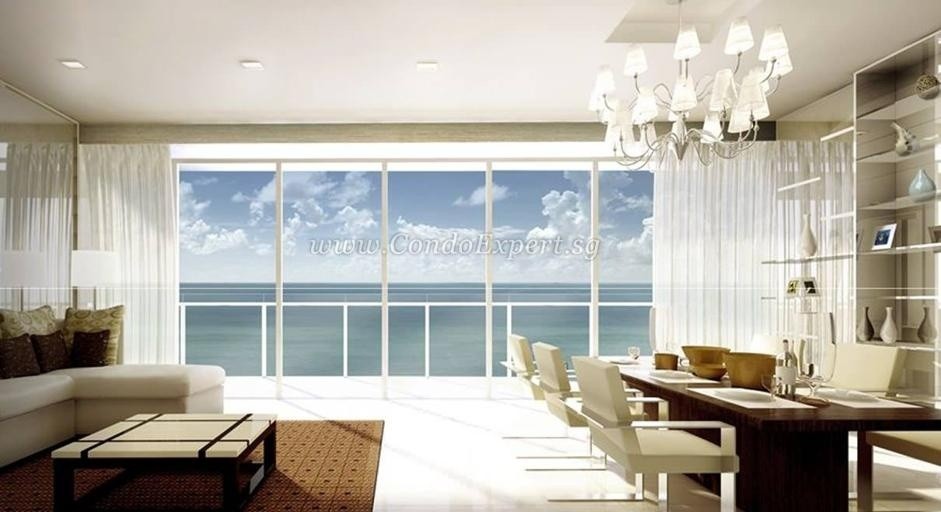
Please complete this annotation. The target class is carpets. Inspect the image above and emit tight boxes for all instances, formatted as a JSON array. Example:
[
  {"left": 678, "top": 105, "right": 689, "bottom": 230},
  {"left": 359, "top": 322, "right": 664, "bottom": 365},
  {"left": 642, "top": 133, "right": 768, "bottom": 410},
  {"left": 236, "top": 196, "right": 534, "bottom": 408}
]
[{"left": 0, "top": 418, "right": 385, "bottom": 512}]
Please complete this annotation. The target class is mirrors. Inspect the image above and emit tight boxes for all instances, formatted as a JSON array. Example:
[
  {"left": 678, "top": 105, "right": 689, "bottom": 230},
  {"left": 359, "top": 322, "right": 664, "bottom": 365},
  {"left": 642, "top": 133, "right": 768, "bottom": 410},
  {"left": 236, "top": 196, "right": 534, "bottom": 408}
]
[{"left": 0, "top": 76, "right": 80, "bottom": 317}]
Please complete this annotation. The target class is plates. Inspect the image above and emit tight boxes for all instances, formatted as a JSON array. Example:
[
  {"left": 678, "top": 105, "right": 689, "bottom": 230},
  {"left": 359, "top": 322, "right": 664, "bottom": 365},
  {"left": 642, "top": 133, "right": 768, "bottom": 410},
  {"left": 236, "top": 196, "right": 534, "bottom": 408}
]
[
  {"left": 648, "top": 370, "right": 694, "bottom": 380},
  {"left": 712, "top": 389, "right": 776, "bottom": 402},
  {"left": 607, "top": 357, "right": 640, "bottom": 365},
  {"left": 812, "top": 388, "right": 878, "bottom": 402}
]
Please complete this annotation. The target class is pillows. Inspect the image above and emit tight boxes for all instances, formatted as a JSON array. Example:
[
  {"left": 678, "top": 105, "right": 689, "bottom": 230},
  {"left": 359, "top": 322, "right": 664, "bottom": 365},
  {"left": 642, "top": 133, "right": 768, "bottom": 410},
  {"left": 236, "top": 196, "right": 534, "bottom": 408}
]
[{"left": 0, "top": 304, "right": 120, "bottom": 381}]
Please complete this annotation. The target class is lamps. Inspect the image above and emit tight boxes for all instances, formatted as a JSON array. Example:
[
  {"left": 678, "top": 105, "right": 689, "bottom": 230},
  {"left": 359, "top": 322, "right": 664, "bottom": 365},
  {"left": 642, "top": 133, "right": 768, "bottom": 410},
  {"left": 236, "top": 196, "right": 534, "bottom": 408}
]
[
  {"left": 0, "top": 247, "right": 51, "bottom": 311},
  {"left": 68, "top": 251, "right": 126, "bottom": 308},
  {"left": 589, "top": 1, "right": 795, "bottom": 173}
]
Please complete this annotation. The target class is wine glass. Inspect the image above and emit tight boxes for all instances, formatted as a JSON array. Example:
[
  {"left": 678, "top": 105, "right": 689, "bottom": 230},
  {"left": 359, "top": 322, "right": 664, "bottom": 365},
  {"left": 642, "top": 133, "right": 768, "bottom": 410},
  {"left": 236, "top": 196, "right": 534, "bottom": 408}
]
[{"left": 797, "top": 311, "right": 839, "bottom": 405}]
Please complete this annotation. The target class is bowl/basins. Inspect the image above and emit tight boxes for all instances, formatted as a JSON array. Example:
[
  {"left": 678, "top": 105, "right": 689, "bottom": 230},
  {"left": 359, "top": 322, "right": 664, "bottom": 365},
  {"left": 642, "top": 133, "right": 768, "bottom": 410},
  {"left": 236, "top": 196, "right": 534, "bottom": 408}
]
[{"left": 690, "top": 366, "right": 729, "bottom": 380}]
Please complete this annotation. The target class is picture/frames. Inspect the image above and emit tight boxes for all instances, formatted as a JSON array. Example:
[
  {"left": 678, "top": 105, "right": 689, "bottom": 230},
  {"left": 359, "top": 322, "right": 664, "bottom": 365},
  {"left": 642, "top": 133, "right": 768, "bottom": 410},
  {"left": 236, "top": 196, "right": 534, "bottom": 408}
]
[
  {"left": 870, "top": 223, "right": 898, "bottom": 252},
  {"left": 787, "top": 279, "right": 802, "bottom": 295}
]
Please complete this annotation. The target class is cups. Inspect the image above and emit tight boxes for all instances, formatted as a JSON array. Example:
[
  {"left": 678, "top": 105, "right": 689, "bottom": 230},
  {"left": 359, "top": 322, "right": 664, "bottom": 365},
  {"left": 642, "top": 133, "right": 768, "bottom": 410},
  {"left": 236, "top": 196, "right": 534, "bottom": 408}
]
[
  {"left": 655, "top": 353, "right": 679, "bottom": 370},
  {"left": 628, "top": 347, "right": 640, "bottom": 360}
]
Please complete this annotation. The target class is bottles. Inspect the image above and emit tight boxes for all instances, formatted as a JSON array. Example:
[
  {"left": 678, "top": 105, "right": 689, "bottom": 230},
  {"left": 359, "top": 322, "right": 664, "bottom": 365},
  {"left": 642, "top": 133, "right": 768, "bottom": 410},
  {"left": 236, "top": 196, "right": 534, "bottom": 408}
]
[
  {"left": 917, "top": 307, "right": 939, "bottom": 346},
  {"left": 909, "top": 170, "right": 937, "bottom": 204},
  {"left": 775, "top": 339, "right": 796, "bottom": 398},
  {"left": 799, "top": 213, "right": 818, "bottom": 257},
  {"left": 855, "top": 306, "right": 874, "bottom": 343},
  {"left": 879, "top": 307, "right": 898, "bottom": 345}
]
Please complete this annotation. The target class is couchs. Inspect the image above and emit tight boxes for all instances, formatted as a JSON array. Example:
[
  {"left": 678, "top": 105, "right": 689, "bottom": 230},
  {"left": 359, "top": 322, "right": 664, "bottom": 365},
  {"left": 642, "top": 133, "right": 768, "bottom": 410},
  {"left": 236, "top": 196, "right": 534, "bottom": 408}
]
[{"left": 0, "top": 319, "right": 226, "bottom": 470}]
